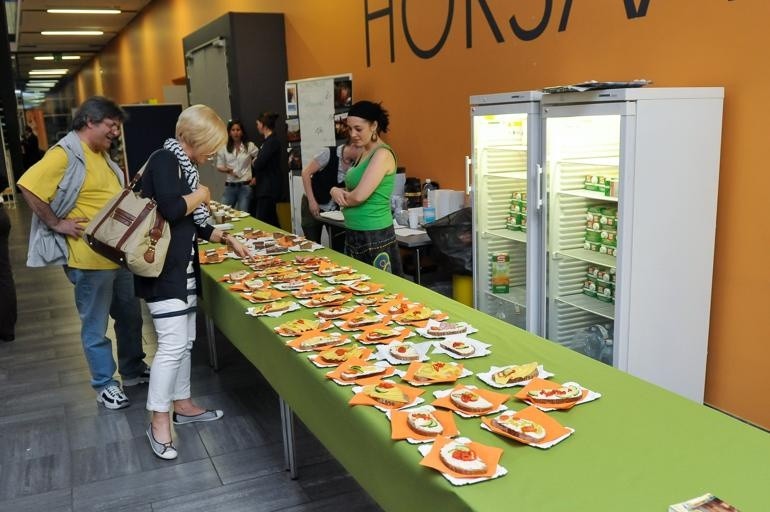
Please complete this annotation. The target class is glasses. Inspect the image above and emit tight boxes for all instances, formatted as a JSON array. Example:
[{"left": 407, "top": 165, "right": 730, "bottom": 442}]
[{"left": 101, "top": 119, "right": 120, "bottom": 130}]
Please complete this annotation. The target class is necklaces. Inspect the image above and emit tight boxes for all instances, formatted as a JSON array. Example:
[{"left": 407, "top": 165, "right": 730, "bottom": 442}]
[{"left": 341, "top": 144, "right": 352, "bottom": 165}]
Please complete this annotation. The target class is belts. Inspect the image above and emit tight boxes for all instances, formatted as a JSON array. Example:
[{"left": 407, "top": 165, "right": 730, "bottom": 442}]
[{"left": 225, "top": 180, "right": 250, "bottom": 188}]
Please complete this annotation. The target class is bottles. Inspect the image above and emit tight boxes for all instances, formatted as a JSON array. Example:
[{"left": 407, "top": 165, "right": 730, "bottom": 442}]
[{"left": 422, "top": 179, "right": 433, "bottom": 207}]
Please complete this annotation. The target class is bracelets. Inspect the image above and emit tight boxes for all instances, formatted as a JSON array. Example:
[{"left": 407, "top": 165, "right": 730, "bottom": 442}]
[{"left": 220, "top": 232, "right": 229, "bottom": 245}]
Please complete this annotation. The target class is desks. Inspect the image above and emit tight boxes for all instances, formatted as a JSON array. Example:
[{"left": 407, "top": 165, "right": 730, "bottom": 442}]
[
  {"left": 195, "top": 198, "right": 770, "bottom": 512},
  {"left": 317, "top": 205, "right": 431, "bottom": 284}
]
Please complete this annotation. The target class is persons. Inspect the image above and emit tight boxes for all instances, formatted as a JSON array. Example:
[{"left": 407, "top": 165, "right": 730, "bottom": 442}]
[
  {"left": 20, "top": 126, "right": 38, "bottom": 165},
  {"left": 217, "top": 120, "right": 257, "bottom": 212},
  {"left": 330, "top": 100, "right": 403, "bottom": 277},
  {"left": 133, "top": 103, "right": 257, "bottom": 460},
  {"left": 251, "top": 111, "right": 283, "bottom": 221},
  {"left": 16, "top": 96, "right": 151, "bottom": 408},
  {"left": 301, "top": 136, "right": 365, "bottom": 252}
]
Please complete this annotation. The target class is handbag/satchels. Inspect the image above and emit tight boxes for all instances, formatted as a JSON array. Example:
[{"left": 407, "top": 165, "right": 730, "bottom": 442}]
[{"left": 81, "top": 148, "right": 182, "bottom": 278}]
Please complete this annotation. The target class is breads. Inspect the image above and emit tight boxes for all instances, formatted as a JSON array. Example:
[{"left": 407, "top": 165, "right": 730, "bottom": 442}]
[
  {"left": 300, "top": 335, "right": 343, "bottom": 348},
  {"left": 318, "top": 307, "right": 354, "bottom": 316},
  {"left": 300, "top": 241, "right": 312, "bottom": 249},
  {"left": 402, "top": 309, "right": 432, "bottom": 322},
  {"left": 491, "top": 366, "right": 539, "bottom": 382},
  {"left": 283, "top": 319, "right": 316, "bottom": 332},
  {"left": 229, "top": 255, "right": 334, "bottom": 291},
  {"left": 341, "top": 365, "right": 386, "bottom": 380},
  {"left": 321, "top": 348, "right": 362, "bottom": 363},
  {"left": 335, "top": 274, "right": 353, "bottom": 282},
  {"left": 252, "top": 230, "right": 263, "bottom": 237},
  {"left": 255, "top": 244, "right": 264, "bottom": 250},
  {"left": 367, "top": 329, "right": 401, "bottom": 339},
  {"left": 254, "top": 293, "right": 272, "bottom": 300},
  {"left": 210, "top": 201, "right": 241, "bottom": 217},
  {"left": 265, "top": 243, "right": 274, "bottom": 247},
  {"left": 349, "top": 315, "right": 379, "bottom": 326},
  {"left": 390, "top": 345, "right": 419, "bottom": 360},
  {"left": 390, "top": 303, "right": 409, "bottom": 315},
  {"left": 491, "top": 415, "right": 546, "bottom": 442},
  {"left": 440, "top": 440, "right": 487, "bottom": 474},
  {"left": 293, "top": 238, "right": 303, "bottom": 245},
  {"left": 350, "top": 283, "right": 370, "bottom": 293},
  {"left": 273, "top": 233, "right": 284, "bottom": 239},
  {"left": 427, "top": 324, "right": 467, "bottom": 336},
  {"left": 207, "top": 252, "right": 218, "bottom": 260},
  {"left": 267, "top": 246, "right": 279, "bottom": 253},
  {"left": 369, "top": 383, "right": 407, "bottom": 406},
  {"left": 243, "top": 228, "right": 253, "bottom": 235},
  {"left": 362, "top": 296, "right": 377, "bottom": 305},
  {"left": 413, "top": 361, "right": 465, "bottom": 386},
  {"left": 450, "top": 388, "right": 494, "bottom": 412},
  {"left": 254, "top": 302, "right": 289, "bottom": 314},
  {"left": 527, "top": 386, "right": 583, "bottom": 403},
  {"left": 440, "top": 340, "right": 475, "bottom": 356},
  {"left": 312, "top": 294, "right": 345, "bottom": 304},
  {"left": 286, "top": 235, "right": 296, "bottom": 242},
  {"left": 205, "top": 249, "right": 215, "bottom": 256},
  {"left": 407, "top": 409, "right": 444, "bottom": 436}
]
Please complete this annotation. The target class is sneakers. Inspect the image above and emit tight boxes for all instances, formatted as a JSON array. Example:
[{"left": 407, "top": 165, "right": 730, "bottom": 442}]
[
  {"left": 96, "top": 383, "right": 130, "bottom": 410},
  {"left": 145, "top": 422, "right": 178, "bottom": 459},
  {"left": 172, "top": 409, "right": 224, "bottom": 425},
  {"left": 121, "top": 365, "right": 151, "bottom": 387}
]
